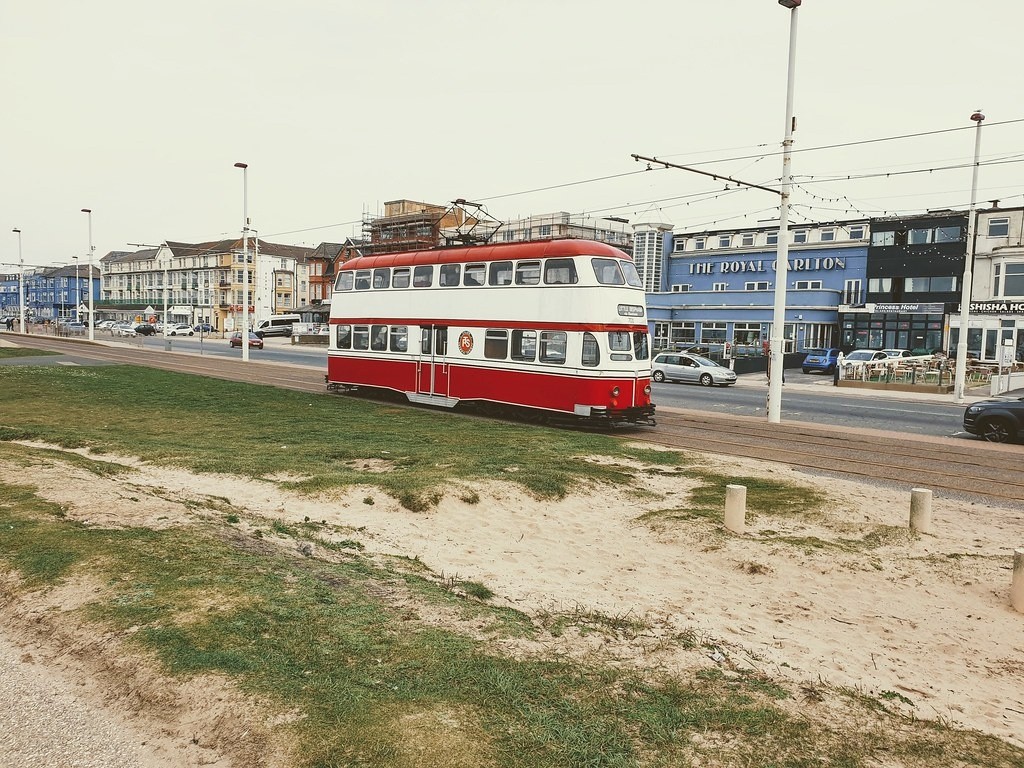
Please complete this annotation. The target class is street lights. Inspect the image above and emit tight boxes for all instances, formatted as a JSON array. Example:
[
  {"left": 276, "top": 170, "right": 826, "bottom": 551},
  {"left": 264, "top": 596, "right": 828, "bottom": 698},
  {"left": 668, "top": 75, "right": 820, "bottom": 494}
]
[
  {"left": 952, "top": 107, "right": 988, "bottom": 399},
  {"left": 233, "top": 162, "right": 249, "bottom": 361},
  {"left": 455, "top": 198, "right": 468, "bottom": 235},
  {"left": 250, "top": 229, "right": 259, "bottom": 330},
  {"left": 71, "top": 255, "right": 79, "bottom": 320},
  {"left": 765, "top": 0, "right": 804, "bottom": 424},
  {"left": 81, "top": 209, "right": 95, "bottom": 342},
  {"left": 12, "top": 229, "right": 25, "bottom": 333}
]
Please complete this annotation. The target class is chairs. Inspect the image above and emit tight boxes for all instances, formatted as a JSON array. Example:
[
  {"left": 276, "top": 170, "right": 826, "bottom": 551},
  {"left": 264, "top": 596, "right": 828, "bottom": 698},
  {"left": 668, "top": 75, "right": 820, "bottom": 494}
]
[{"left": 846, "top": 358, "right": 1019, "bottom": 384}]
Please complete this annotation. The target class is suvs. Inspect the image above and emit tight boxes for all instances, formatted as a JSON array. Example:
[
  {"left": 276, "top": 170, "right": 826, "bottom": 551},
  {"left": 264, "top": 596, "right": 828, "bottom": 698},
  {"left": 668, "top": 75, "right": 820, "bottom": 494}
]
[
  {"left": 111, "top": 324, "right": 137, "bottom": 338},
  {"left": 649, "top": 351, "right": 739, "bottom": 387},
  {"left": 135, "top": 324, "right": 156, "bottom": 336}
]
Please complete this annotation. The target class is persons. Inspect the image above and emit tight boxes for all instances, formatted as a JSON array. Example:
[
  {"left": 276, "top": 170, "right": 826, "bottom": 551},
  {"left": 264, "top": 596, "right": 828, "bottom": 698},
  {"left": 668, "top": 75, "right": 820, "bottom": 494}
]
[{"left": 5, "top": 318, "right": 17, "bottom": 331}]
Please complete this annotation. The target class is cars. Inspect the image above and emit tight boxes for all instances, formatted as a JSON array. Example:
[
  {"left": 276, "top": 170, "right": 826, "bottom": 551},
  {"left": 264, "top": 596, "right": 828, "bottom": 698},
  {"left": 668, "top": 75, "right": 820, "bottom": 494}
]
[
  {"left": 963, "top": 393, "right": 1024, "bottom": 446},
  {"left": 163, "top": 324, "right": 194, "bottom": 337},
  {"left": 230, "top": 331, "right": 263, "bottom": 350},
  {"left": 801, "top": 347, "right": 914, "bottom": 380},
  {"left": 0, "top": 315, "right": 215, "bottom": 333}
]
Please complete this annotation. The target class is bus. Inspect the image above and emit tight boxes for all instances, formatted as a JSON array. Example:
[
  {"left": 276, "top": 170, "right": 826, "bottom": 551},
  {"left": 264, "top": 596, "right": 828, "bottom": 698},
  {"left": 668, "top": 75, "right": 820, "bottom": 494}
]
[{"left": 321, "top": 235, "right": 658, "bottom": 431}]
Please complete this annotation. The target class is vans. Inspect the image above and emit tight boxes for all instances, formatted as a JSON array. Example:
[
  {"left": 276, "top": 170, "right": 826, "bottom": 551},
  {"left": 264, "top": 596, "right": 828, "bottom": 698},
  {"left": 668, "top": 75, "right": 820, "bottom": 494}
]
[{"left": 253, "top": 313, "right": 303, "bottom": 336}]
